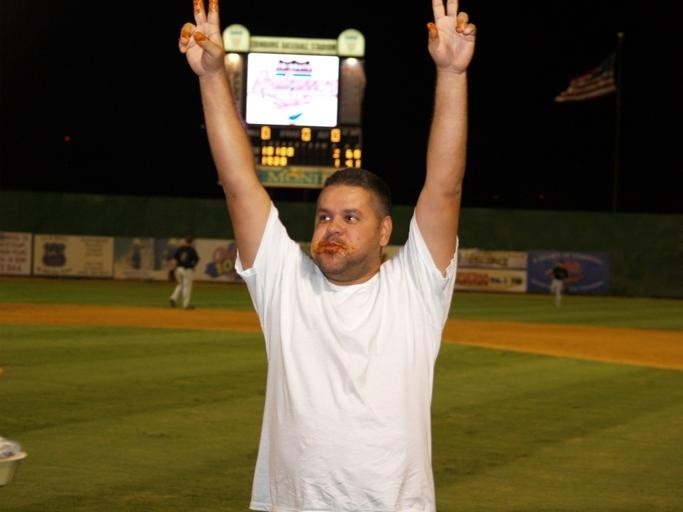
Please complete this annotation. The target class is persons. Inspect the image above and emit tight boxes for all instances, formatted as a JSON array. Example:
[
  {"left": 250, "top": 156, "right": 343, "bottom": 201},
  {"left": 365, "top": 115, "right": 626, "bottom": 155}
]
[
  {"left": 543, "top": 259, "right": 570, "bottom": 307},
  {"left": 173, "top": 0, "right": 485, "bottom": 511},
  {"left": 166, "top": 232, "right": 200, "bottom": 314}
]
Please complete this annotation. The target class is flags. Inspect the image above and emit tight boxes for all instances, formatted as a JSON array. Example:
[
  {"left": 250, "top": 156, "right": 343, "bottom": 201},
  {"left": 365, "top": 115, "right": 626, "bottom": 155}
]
[{"left": 551, "top": 50, "right": 621, "bottom": 105}]
